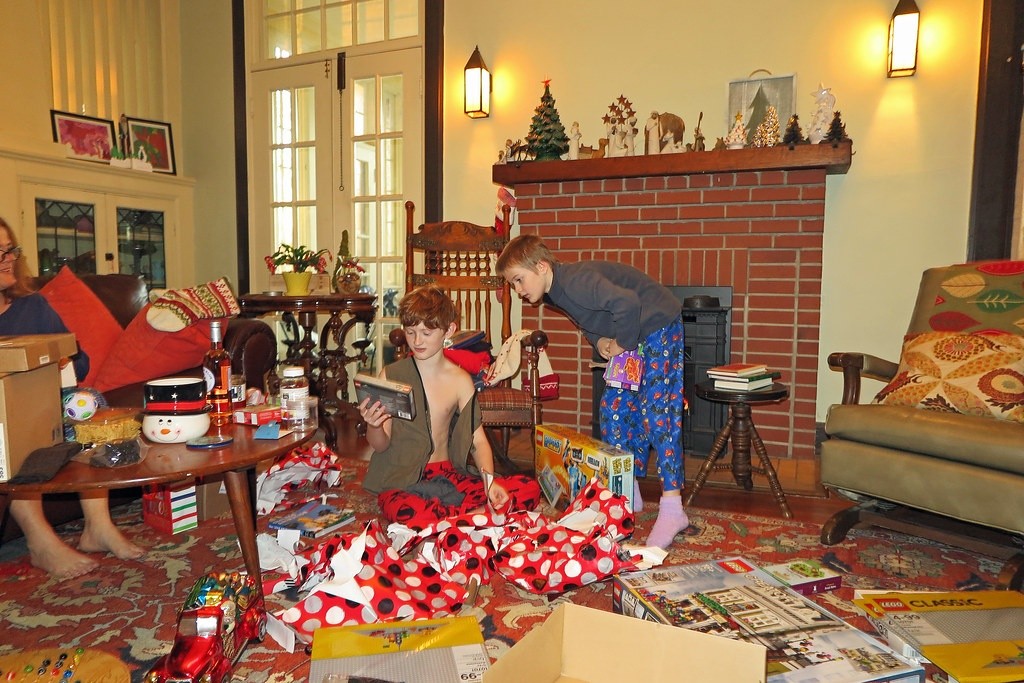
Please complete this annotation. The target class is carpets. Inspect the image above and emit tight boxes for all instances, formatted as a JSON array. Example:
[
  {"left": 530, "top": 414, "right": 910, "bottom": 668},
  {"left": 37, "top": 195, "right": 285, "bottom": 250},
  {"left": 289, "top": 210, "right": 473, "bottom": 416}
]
[{"left": 0, "top": 455, "right": 1007, "bottom": 683}]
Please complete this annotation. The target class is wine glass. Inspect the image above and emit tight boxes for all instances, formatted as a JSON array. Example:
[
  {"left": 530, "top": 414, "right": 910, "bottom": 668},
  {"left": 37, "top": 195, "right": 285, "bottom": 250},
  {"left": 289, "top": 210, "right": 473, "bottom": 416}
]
[{"left": 205, "top": 402, "right": 231, "bottom": 435}]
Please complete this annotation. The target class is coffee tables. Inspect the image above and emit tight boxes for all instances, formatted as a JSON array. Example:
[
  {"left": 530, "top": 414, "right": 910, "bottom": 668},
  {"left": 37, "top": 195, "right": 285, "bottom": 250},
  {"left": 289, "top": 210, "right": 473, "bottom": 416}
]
[{"left": 0, "top": 422, "right": 318, "bottom": 615}]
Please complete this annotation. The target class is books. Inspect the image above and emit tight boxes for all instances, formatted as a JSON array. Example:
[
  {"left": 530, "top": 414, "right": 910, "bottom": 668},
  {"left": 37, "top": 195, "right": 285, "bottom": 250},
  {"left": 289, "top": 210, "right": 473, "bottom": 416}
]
[
  {"left": 705, "top": 362, "right": 781, "bottom": 391},
  {"left": 270, "top": 501, "right": 356, "bottom": 539},
  {"left": 603, "top": 344, "right": 645, "bottom": 391}
]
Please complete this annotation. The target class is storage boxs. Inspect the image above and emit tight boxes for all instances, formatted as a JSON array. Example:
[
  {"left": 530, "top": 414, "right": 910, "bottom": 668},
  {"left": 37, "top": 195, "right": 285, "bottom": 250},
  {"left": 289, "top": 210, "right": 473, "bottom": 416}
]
[
  {"left": 354, "top": 373, "right": 418, "bottom": 422},
  {"left": 610, "top": 555, "right": 926, "bottom": 683},
  {"left": 854, "top": 589, "right": 953, "bottom": 665},
  {"left": 282, "top": 272, "right": 313, "bottom": 296},
  {"left": 232, "top": 404, "right": 281, "bottom": 426},
  {"left": 483, "top": 601, "right": 768, "bottom": 683},
  {"left": 533, "top": 424, "right": 635, "bottom": 515},
  {"left": 763, "top": 559, "right": 842, "bottom": 596},
  {"left": 142, "top": 484, "right": 199, "bottom": 537},
  {"left": 308, "top": 274, "right": 332, "bottom": 297},
  {"left": 195, "top": 481, "right": 230, "bottom": 522},
  {"left": 604, "top": 341, "right": 646, "bottom": 392},
  {"left": 0, "top": 333, "right": 79, "bottom": 374},
  {"left": 0, "top": 362, "right": 65, "bottom": 485}
]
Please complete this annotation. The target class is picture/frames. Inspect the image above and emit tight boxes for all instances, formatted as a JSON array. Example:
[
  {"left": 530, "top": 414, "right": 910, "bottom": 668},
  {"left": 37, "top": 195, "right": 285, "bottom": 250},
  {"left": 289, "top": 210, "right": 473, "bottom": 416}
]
[
  {"left": 119, "top": 117, "right": 177, "bottom": 175},
  {"left": 727, "top": 72, "right": 797, "bottom": 144},
  {"left": 50, "top": 109, "right": 117, "bottom": 165}
]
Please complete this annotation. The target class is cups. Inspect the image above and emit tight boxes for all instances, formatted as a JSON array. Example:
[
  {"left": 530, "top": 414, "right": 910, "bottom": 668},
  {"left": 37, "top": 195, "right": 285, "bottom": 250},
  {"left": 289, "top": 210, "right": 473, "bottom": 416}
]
[
  {"left": 309, "top": 397, "right": 318, "bottom": 428},
  {"left": 232, "top": 375, "right": 246, "bottom": 408}
]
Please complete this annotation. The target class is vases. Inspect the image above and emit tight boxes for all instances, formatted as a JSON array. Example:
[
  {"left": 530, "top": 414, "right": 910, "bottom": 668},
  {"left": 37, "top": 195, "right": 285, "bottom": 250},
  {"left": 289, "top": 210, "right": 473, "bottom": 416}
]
[{"left": 336, "top": 256, "right": 364, "bottom": 294}]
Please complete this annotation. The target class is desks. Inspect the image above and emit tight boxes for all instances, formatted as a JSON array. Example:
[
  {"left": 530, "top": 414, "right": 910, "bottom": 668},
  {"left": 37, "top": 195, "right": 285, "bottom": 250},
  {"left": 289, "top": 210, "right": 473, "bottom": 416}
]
[{"left": 682, "top": 379, "right": 795, "bottom": 519}]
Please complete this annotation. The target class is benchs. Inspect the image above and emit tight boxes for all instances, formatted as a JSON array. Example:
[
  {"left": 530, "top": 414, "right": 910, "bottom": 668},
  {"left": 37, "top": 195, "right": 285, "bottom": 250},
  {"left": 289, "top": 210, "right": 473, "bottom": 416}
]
[{"left": 818, "top": 260, "right": 1024, "bottom": 593}]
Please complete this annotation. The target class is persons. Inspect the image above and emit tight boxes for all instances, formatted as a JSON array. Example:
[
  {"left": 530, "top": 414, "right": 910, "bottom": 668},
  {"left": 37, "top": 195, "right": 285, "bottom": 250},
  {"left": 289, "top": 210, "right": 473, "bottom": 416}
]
[
  {"left": 0, "top": 218, "right": 143, "bottom": 577},
  {"left": 358, "top": 285, "right": 540, "bottom": 527},
  {"left": 496, "top": 234, "right": 690, "bottom": 547}
]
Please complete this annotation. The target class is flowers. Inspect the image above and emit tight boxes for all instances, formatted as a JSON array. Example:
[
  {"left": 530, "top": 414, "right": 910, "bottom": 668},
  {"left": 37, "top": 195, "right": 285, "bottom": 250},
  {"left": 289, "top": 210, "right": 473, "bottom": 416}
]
[{"left": 263, "top": 243, "right": 333, "bottom": 275}]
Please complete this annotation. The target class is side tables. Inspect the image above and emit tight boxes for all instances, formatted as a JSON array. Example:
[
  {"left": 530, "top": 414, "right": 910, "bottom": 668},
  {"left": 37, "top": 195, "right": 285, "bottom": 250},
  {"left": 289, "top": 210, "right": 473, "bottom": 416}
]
[{"left": 240, "top": 292, "right": 379, "bottom": 451}]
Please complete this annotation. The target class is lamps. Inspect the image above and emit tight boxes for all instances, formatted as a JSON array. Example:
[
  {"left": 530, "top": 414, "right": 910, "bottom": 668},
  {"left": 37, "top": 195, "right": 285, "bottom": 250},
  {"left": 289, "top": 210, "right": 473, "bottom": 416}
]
[
  {"left": 886, "top": 0, "right": 920, "bottom": 79},
  {"left": 464, "top": 43, "right": 493, "bottom": 119}
]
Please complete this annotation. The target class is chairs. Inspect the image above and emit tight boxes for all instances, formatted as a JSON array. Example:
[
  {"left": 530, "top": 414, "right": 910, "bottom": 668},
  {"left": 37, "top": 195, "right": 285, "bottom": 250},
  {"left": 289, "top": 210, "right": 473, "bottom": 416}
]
[{"left": 403, "top": 201, "right": 543, "bottom": 477}]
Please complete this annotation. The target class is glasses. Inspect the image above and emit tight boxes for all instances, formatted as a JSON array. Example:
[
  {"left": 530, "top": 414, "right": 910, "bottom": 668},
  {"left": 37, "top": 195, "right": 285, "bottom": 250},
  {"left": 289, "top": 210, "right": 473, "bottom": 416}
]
[{"left": 0, "top": 245, "right": 21, "bottom": 264}]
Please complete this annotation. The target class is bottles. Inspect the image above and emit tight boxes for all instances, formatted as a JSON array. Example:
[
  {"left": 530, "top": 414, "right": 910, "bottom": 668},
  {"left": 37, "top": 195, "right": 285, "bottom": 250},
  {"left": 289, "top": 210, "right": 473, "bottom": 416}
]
[
  {"left": 203, "top": 322, "right": 231, "bottom": 415},
  {"left": 336, "top": 256, "right": 361, "bottom": 294},
  {"left": 280, "top": 367, "right": 310, "bottom": 430}
]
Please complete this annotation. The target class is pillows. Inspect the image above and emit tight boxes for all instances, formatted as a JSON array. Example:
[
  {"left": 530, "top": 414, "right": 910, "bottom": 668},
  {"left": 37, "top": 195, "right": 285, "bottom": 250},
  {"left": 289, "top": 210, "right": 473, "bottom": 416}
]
[
  {"left": 869, "top": 329, "right": 1024, "bottom": 423},
  {"left": 37, "top": 264, "right": 126, "bottom": 390},
  {"left": 93, "top": 301, "right": 229, "bottom": 394},
  {"left": 409, "top": 350, "right": 492, "bottom": 378}
]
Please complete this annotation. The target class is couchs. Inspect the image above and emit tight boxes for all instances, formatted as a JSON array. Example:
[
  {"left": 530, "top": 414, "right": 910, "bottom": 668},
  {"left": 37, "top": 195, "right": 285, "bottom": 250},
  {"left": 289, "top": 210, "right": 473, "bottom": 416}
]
[{"left": 0, "top": 274, "right": 279, "bottom": 545}]
